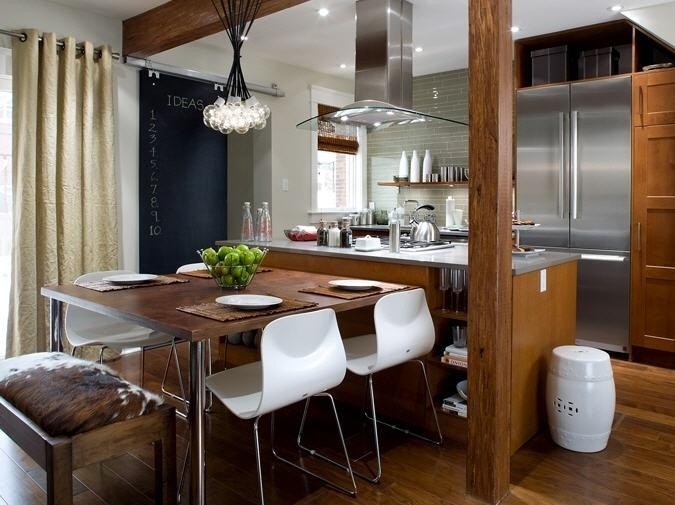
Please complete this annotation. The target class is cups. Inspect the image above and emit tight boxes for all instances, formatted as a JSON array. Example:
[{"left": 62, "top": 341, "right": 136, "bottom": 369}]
[{"left": 452, "top": 326, "right": 467, "bottom": 348}]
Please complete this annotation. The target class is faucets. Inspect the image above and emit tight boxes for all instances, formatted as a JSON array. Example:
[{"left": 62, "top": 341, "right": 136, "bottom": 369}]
[{"left": 403, "top": 199, "right": 420, "bottom": 209}]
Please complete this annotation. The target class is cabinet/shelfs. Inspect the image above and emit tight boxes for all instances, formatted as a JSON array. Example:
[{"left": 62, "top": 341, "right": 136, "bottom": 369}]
[
  {"left": 426, "top": 266, "right": 470, "bottom": 447},
  {"left": 631, "top": 68, "right": 675, "bottom": 353}
]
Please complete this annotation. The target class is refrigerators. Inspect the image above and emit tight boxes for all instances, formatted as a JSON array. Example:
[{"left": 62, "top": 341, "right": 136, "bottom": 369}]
[{"left": 516, "top": 75, "right": 633, "bottom": 355}]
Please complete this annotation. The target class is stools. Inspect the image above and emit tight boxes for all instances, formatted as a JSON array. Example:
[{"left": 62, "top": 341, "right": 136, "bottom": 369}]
[{"left": 0, "top": 350, "right": 176, "bottom": 505}]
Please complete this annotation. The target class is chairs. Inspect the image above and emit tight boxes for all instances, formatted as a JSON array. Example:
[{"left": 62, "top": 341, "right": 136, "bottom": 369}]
[
  {"left": 164, "top": 263, "right": 263, "bottom": 411},
  {"left": 64, "top": 271, "right": 190, "bottom": 425},
  {"left": 206, "top": 309, "right": 359, "bottom": 504},
  {"left": 297, "top": 289, "right": 444, "bottom": 485}
]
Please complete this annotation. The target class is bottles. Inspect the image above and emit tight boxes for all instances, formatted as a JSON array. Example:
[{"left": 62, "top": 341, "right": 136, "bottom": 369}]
[
  {"left": 388, "top": 207, "right": 404, "bottom": 254},
  {"left": 445, "top": 196, "right": 462, "bottom": 227},
  {"left": 399, "top": 149, "right": 469, "bottom": 183},
  {"left": 239, "top": 201, "right": 272, "bottom": 242},
  {"left": 317, "top": 208, "right": 375, "bottom": 248}
]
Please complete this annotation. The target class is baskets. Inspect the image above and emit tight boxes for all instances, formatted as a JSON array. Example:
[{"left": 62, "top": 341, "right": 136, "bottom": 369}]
[{"left": 195, "top": 247, "right": 270, "bottom": 289}]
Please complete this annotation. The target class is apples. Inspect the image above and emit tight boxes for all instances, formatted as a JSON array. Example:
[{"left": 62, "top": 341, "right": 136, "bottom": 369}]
[{"left": 201, "top": 244, "right": 264, "bottom": 285}]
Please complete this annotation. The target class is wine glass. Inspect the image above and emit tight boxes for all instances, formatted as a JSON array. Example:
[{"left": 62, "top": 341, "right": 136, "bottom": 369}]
[{"left": 438, "top": 268, "right": 468, "bottom": 315}]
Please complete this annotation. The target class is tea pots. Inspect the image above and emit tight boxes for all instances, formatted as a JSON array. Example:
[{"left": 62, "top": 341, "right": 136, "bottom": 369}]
[{"left": 409, "top": 205, "right": 440, "bottom": 241}]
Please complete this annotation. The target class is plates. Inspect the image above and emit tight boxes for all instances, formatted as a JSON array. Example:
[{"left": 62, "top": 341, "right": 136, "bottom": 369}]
[
  {"left": 329, "top": 280, "right": 380, "bottom": 291},
  {"left": 215, "top": 294, "right": 283, "bottom": 309},
  {"left": 456, "top": 379, "right": 467, "bottom": 400},
  {"left": 103, "top": 274, "right": 156, "bottom": 284},
  {"left": 441, "top": 227, "right": 468, "bottom": 232}
]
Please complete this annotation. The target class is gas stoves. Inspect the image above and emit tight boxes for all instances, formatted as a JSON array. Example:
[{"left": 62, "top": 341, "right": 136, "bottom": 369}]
[{"left": 374, "top": 235, "right": 454, "bottom": 250}]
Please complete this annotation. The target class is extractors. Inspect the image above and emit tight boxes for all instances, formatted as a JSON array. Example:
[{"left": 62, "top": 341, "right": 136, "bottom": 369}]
[{"left": 296, "top": 1, "right": 469, "bottom": 132}]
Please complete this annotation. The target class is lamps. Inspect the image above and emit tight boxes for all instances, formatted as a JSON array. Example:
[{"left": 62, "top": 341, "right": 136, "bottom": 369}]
[{"left": 203, "top": 0, "right": 270, "bottom": 134}]
